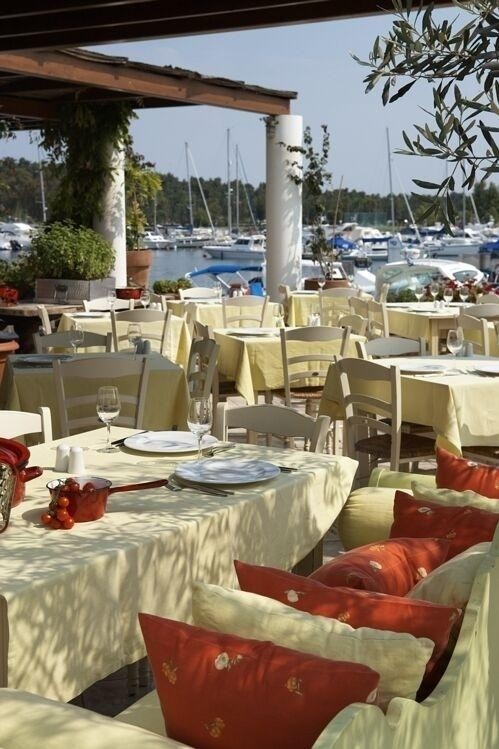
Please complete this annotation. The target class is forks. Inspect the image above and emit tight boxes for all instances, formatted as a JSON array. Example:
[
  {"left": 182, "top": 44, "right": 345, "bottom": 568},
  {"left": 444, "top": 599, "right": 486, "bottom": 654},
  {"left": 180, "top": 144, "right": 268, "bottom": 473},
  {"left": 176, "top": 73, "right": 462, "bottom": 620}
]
[
  {"left": 201, "top": 441, "right": 238, "bottom": 458},
  {"left": 165, "top": 482, "right": 228, "bottom": 498}
]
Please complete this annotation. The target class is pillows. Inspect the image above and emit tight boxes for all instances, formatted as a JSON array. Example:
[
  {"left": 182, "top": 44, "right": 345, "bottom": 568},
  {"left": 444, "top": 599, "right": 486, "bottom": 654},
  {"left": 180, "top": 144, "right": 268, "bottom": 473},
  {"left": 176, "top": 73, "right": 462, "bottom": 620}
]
[
  {"left": 139, "top": 560, "right": 458, "bottom": 742},
  {"left": 319, "top": 450, "right": 499, "bottom": 606}
]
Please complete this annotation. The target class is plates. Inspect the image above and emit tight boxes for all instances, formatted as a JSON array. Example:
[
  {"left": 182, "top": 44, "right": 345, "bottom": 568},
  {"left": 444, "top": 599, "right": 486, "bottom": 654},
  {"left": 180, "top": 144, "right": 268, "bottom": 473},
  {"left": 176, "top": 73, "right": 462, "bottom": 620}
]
[
  {"left": 394, "top": 361, "right": 445, "bottom": 375},
  {"left": 174, "top": 459, "right": 280, "bottom": 487},
  {"left": 386, "top": 302, "right": 410, "bottom": 308},
  {"left": 474, "top": 364, "right": 499, "bottom": 376},
  {"left": 15, "top": 354, "right": 73, "bottom": 366},
  {"left": 227, "top": 328, "right": 272, "bottom": 337},
  {"left": 122, "top": 432, "right": 220, "bottom": 454}
]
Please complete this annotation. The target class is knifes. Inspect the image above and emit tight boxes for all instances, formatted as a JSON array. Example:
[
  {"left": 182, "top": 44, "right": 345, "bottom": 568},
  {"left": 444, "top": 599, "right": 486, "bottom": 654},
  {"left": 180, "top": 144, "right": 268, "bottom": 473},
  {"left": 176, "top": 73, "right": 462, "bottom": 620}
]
[{"left": 111, "top": 430, "right": 150, "bottom": 445}]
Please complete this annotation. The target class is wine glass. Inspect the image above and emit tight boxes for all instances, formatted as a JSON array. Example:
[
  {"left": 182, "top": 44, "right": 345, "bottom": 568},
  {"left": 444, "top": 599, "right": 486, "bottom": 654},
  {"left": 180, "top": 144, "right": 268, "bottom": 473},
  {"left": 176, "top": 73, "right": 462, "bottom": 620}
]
[
  {"left": 412, "top": 284, "right": 470, "bottom": 311},
  {"left": 270, "top": 272, "right": 355, "bottom": 338},
  {"left": 446, "top": 330, "right": 465, "bottom": 371},
  {"left": 65, "top": 288, "right": 151, "bottom": 360},
  {"left": 185, "top": 395, "right": 215, "bottom": 459},
  {"left": 93, "top": 384, "right": 123, "bottom": 454},
  {"left": 209, "top": 281, "right": 251, "bottom": 306}
]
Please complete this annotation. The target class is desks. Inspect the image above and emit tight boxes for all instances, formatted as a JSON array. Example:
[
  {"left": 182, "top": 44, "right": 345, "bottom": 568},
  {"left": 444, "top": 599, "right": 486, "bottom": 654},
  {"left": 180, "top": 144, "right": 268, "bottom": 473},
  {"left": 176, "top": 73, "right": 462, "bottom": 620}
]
[
  {"left": 4, "top": 350, "right": 191, "bottom": 435},
  {"left": 317, "top": 354, "right": 497, "bottom": 467},
  {"left": 2, "top": 426, "right": 358, "bottom": 703}
]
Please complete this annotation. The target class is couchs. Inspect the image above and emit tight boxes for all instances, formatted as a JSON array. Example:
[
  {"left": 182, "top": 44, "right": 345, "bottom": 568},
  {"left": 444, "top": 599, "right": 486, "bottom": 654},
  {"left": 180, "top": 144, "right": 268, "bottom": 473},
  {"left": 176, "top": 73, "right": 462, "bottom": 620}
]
[{"left": 0, "top": 469, "right": 495, "bottom": 744}]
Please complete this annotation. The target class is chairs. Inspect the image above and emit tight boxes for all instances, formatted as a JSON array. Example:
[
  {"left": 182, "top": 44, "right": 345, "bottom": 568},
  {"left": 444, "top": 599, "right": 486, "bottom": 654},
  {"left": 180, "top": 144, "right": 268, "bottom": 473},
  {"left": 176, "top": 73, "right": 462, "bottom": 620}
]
[
  {"left": 265, "top": 326, "right": 349, "bottom": 411},
  {"left": 213, "top": 402, "right": 331, "bottom": 454},
  {"left": 335, "top": 356, "right": 437, "bottom": 472},
  {"left": 52, "top": 356, "right": 148, "bottom": 437},
  {"left": 185, "top": 339, "right": 219, "bottom": 426},
  {"left": 6, "top": 288, "right": 499, "bottom": 363},
  {"left": 2, "top": 407, "right": 52, "bottom": 449}
]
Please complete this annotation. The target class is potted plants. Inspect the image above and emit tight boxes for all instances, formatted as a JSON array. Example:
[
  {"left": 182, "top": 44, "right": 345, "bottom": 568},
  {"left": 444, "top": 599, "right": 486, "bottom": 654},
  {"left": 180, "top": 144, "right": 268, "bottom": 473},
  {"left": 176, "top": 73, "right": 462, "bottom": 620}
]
[
  {"left": 276, "top": 124, "right": 350, "bottom": 289},
  {"left": 122, "top": 147, "right": 163, "bottom": 289},
  {"left": 25, "top": 217, "right": 117, "bottom": 304}
]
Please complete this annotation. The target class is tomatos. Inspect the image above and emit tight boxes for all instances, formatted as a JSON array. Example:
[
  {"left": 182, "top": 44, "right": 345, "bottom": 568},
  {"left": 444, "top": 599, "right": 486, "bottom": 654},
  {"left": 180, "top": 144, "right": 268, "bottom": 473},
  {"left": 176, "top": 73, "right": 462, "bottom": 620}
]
[{"left": 42, "top": 478, "right": 96, "bottom": 529}]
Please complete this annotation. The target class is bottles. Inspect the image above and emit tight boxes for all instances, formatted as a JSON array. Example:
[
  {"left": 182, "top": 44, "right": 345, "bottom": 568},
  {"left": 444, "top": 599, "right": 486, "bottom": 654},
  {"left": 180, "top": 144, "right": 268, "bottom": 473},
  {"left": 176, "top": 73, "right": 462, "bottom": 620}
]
[{"left": 53, "top": 444, "right": 87, "bottom": 474}]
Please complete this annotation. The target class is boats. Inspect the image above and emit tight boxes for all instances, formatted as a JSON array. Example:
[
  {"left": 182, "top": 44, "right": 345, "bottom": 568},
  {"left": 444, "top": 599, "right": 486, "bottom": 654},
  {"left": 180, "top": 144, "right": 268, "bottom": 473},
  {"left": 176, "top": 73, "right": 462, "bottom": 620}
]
[
  {"left": 356, "top": 251, "right": 492, "bottom": 305},
  {"left": 171, "top": 256, "right": 380, "bottom": 304}
]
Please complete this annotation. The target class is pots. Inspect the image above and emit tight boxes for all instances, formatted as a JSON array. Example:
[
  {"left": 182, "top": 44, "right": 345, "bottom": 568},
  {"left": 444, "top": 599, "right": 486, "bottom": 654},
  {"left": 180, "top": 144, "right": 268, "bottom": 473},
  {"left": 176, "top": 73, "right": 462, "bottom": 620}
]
[
  {"left": 44, "top": 476, "right": 167, "bottom": 524},
  {"left": 0, "top": 438, "right": 42, "bottom": 508}
]
